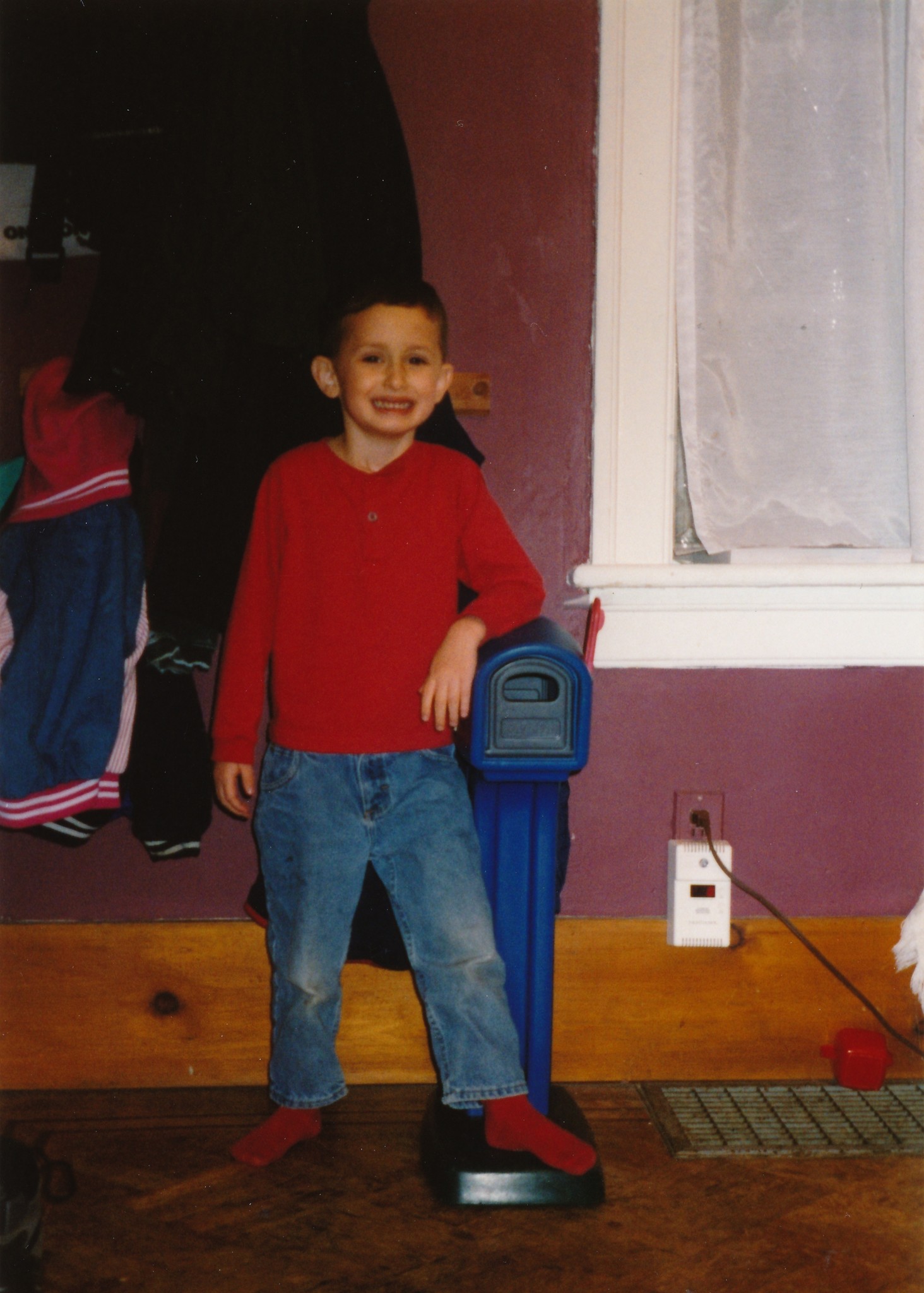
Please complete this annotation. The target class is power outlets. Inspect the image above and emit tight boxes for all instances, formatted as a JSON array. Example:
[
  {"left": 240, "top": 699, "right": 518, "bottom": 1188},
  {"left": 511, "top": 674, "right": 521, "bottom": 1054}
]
[{"left": 676, "top": 792, "right": 724, "bottom": 840}]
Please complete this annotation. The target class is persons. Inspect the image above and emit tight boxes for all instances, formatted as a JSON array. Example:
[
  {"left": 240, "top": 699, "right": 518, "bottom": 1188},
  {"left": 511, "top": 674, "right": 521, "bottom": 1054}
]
[{"left": 212, "top": 273, "right": 598, "bottom": 1175}]
